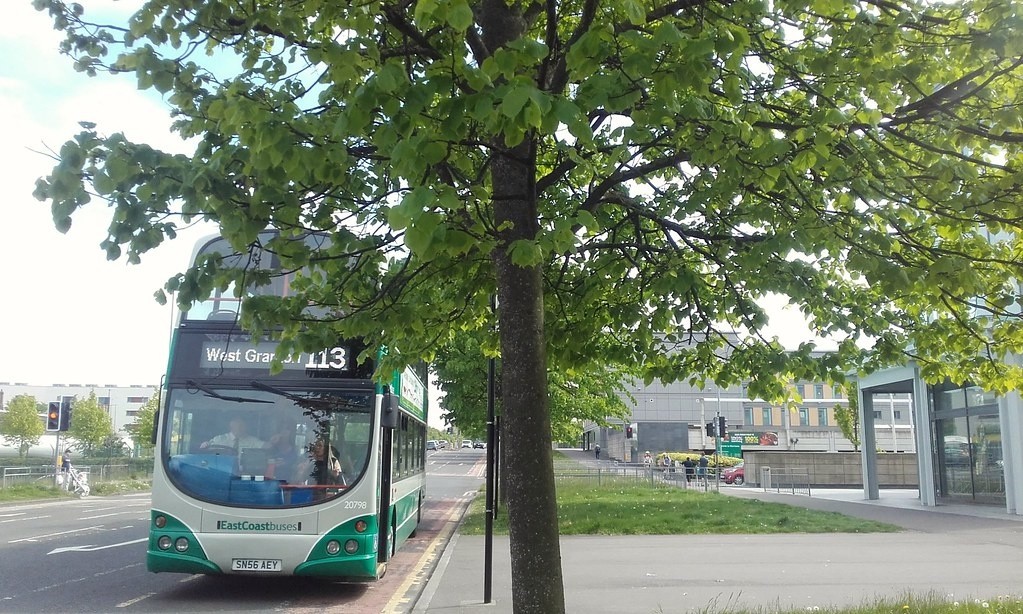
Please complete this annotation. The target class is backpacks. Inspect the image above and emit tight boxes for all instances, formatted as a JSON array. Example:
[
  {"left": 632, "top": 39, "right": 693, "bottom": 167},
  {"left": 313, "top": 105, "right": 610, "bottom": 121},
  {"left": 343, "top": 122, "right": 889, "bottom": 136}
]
[
  {"left": 595, "top": 445, "right": 601, "bottom": 453},
  {"left": 663, "top": 454, "right": 670, "bottom": 466}
]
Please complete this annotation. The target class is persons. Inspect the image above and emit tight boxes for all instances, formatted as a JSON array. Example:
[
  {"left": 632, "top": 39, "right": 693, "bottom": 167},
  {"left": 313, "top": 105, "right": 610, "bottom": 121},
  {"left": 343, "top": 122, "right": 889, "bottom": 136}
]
[
  {"left": 682, "top": 457, "right": 694, "bottom": 482},
  {"left": 698, "top": 451, "right": 709, "bottom": 487},
  {"left": 644, "top": 451, "right": 652, "bottom": 473},
  {"left": 292, "top": 434, "right": 345, "bottom": 485},
  {"left": 663, "top": 454, "right": 671, "bottom": 474},
  {"left": 62, "top": 450, "right": 72, "bottom": 491},
  {"left": 595, "top": 443, "right": 600, "bottom": 459},
  {"left": 199, "top": 415, "right": 281, "bottom": 472}
]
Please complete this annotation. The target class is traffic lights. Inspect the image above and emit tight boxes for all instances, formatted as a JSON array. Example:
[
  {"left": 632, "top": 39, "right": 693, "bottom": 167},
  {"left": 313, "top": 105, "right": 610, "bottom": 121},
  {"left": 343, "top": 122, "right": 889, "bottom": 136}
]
[
  {"left": 46, "top": 401, "right": 60, "bottom": 432},
  {"left": 61, "top": 403, "right": 73, "bottom": 432},
  {"left": 626, "top": 427, "right": 633, "bottom": 439},
  {"left": 720, "top": 417, "right": 729, "bottom": 438}
]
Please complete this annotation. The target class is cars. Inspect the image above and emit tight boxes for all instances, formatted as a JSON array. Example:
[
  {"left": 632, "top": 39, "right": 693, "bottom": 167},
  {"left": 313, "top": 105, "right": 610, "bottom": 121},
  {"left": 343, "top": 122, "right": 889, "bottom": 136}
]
[
  {"left": 474, "top": 440, "right": 485, "bottom": 449},
  {"left": 426, "top": 440, "right": 441, "bottom": 450},
  {"left": 720, "top": 462, "right": 745, "bottom": 486},
  {"left": 462, "top": 440, "right": 473, "bottom": 448},
  {"left": 438, "top": 440, "right": 447, "bottom": 449},
  {"left": 942, "top": 440, "right": 993, "bottom": 466}
]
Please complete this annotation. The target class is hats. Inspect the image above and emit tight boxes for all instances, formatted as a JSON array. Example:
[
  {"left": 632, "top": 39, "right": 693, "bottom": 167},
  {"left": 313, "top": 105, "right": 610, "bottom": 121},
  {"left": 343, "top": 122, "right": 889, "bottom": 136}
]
[
  {"left": 65, "top": 450, "right": 72, "bottom": 453},
  {"left": 645, "top": 451, "right": 650, "bottom": 454}
]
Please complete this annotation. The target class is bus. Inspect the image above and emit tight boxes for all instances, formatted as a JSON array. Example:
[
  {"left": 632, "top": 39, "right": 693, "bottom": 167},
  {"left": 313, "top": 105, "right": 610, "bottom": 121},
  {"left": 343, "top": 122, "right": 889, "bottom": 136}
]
[{"left": 145, "top": 233, "right": 429, "bottom": 582}]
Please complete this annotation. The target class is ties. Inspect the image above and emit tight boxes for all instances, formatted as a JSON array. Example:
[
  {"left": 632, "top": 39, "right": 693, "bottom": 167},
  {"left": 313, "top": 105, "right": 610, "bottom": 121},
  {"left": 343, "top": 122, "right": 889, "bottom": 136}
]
[{"left": 233, "top": 439, "right": 239, "bottom": 450}]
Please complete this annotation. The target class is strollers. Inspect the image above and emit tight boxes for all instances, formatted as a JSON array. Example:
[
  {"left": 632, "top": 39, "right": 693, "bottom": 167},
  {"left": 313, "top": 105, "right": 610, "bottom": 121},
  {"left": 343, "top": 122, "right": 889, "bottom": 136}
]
[{"left": 68, "top": 469, "right": 91, "bottom": 497}]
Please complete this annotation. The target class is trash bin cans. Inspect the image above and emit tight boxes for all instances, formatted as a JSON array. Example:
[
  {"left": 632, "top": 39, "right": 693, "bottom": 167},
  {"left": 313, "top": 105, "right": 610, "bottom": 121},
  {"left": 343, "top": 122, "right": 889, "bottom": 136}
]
[{"left": 696, "top": 465, "right": 706, "bottom": 479}]
